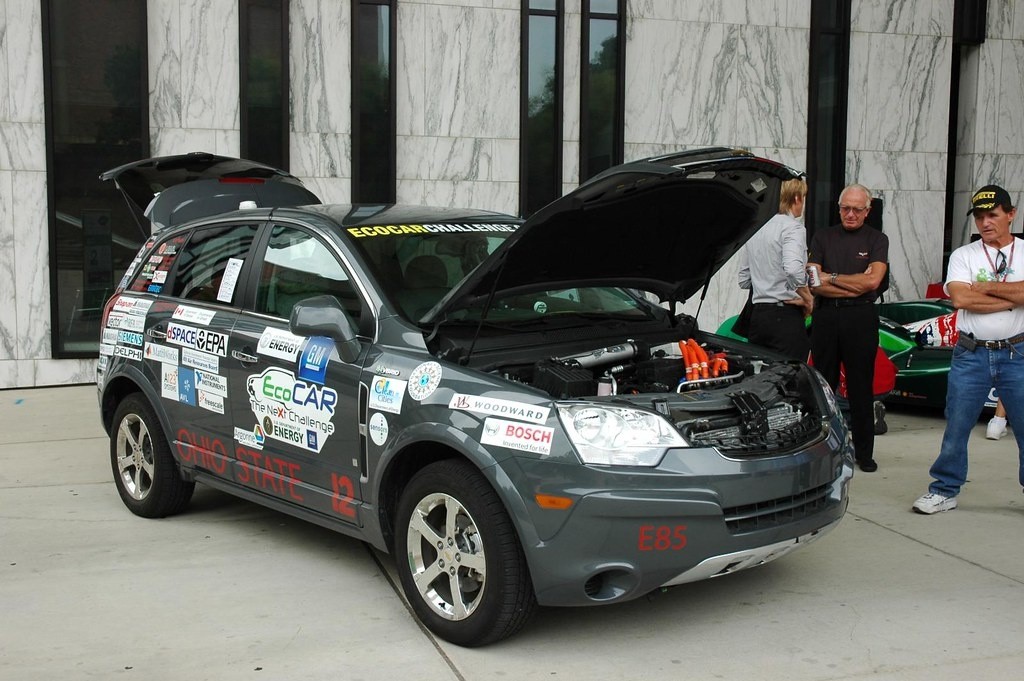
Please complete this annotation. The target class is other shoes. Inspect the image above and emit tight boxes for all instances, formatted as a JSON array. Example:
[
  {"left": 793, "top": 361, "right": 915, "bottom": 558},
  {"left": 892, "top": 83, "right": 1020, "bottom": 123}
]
[
  {"left": 855, "top": 456, "right": 877, "bottom": 472},
  {"left": 872, "top": 401, "right": 887, "bottom": 434}
]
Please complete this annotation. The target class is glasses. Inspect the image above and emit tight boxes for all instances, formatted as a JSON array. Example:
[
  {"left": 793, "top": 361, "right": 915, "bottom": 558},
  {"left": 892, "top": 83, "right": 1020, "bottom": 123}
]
[
  {"left": 994, "top": 249, "right": 1007, "bottom": 275},
  {"left": 839, "top": 204, "right": 868, "bottom": 214}
]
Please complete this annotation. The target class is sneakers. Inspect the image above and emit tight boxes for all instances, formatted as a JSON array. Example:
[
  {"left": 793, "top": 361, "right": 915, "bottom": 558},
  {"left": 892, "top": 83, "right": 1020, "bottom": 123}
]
[
  {"left": 986, "top": 419, "right": 1007, "bottom": 440},
  {"left": 912, "top": 493, "right": 958, "bottom": 514}
]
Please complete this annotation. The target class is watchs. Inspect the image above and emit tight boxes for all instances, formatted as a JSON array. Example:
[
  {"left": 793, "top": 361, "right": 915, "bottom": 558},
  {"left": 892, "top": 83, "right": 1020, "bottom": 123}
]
[{"left": 830, "top": 273, "right": 838, "bottom": 284}]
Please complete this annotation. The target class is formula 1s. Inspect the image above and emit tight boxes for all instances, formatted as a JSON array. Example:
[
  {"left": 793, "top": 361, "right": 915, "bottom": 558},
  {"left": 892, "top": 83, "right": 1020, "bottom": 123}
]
[{"left": 714, "top": 298, "right": 1001, "bottom": 408}]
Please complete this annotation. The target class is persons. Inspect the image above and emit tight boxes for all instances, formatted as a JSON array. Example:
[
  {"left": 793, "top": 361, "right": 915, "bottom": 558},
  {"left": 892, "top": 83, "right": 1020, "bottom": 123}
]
[
  {"left": 807, "top": 185, "right": 890, "bottom": 472},
  {"left": 913, "top": 185, "right": 1024, "bottom": 514},
  {"left": 986, "top": 398, "right": 1007, "bottom": 439},
  {"left": 738, "top": 179, "right": 812, "bottom": 362}
]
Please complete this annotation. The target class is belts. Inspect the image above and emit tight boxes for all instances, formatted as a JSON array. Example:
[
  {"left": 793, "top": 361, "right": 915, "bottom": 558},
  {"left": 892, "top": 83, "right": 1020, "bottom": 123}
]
[
  {"left": 815, "top": 299, "right": 871, "bottom": 307},
  {"left": 754, "top": 301, "right": 793, "bottom": 307},
  {"left": 975, "top": 335, "right": 1024, "bottom": 350}
]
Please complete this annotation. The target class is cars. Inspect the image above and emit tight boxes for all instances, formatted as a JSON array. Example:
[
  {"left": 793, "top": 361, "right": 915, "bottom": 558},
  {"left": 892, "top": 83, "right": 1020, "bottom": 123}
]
[{"left": 95, "top": 148, "right": 856, "bottom": 648}]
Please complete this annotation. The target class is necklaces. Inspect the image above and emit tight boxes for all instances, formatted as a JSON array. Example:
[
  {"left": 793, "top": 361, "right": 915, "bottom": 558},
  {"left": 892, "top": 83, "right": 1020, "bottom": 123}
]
[{"left": 983, "top": 237, "right": 1015, "bottom": 283}]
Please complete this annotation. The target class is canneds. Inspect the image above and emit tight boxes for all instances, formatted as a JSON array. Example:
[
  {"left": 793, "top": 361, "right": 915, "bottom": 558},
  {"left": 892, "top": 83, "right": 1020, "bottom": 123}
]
[{"left": 807, "top": 265, "right": 821, "bottom": 286}]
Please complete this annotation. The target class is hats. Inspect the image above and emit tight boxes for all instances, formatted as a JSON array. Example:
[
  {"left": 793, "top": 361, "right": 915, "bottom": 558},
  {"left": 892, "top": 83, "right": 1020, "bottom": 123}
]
[{"left": 967, "top": 185, "right": 1014, "bottom": 217}]
